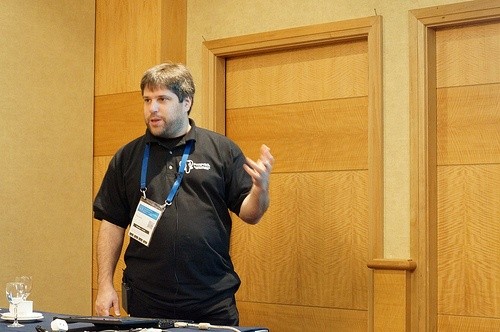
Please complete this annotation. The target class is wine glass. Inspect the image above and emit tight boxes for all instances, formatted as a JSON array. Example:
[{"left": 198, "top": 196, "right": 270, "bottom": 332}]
[
  {"left": 5, "top": 282, "right": 25, "bottom": 328},
  {"left": 16, "top": 276, "right": 32, "bottom": 300}
]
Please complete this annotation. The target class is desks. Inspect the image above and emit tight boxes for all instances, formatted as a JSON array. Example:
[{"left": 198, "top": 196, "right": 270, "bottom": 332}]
[{"left": 0, "top": 307, "right": 269, "bottom": 332}]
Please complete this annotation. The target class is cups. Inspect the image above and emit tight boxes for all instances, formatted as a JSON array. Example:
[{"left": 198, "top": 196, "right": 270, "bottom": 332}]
[{"left": 9, "top": 300, "right": 33, "bottom": 314}]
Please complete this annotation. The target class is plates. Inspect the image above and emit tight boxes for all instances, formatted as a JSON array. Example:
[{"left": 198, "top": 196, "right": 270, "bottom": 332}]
[
  {"left": 0, "top": 316, "right": 45, "bottom": 322},
  {"left": 2, "top": 312, "right": 42, "bottom": 318}
]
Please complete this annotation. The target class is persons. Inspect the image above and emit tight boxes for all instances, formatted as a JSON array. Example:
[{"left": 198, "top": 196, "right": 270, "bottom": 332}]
[{"left": 94, "top": 59, "right": 274, "bottom": 327}]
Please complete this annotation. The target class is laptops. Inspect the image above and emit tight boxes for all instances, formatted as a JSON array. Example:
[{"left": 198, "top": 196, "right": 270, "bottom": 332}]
[{"left": 53, "top": 316, "right": 195, "bottom": 331}]
